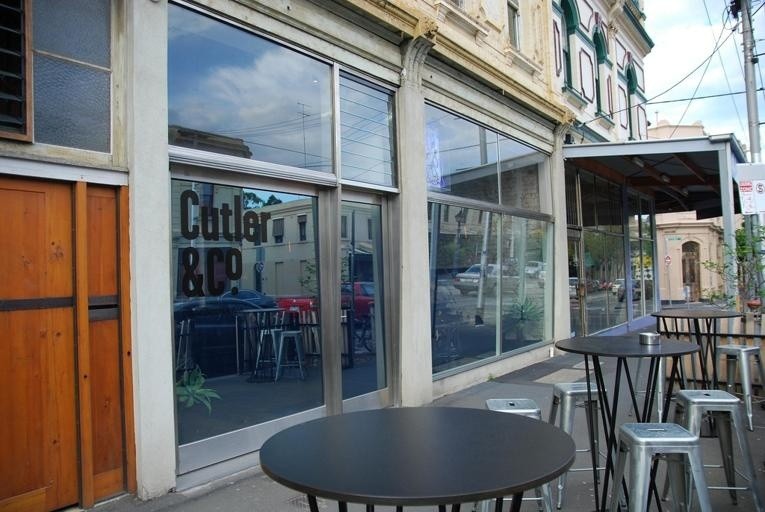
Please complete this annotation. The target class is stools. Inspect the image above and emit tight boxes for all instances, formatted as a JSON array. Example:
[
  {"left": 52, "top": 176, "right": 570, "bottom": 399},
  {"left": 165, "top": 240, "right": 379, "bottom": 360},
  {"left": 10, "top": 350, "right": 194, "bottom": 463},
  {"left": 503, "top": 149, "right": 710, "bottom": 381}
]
[
  {"left": 482, "top": 398, "right": 554, "bottom": 512},
  {"left": 252, "top": 328, "right": 288, "bottom": 382},
  {"left": 628, "top": 336, "right": 688, "bottom": 423},
  {"left": 609, "top": 422, "right": 713, "bottom": 512},
  {"left": 547, "top": 382, "right": 626, "bottom": 510},
  {"left": 274, "top": 331, "right": 308, "bottom": 383},
  {"left": 710, "top": 345, "right": 765, "bottom": 432},
  {"left": 661, "top": 390, "right": 764, "bottom": 512}
]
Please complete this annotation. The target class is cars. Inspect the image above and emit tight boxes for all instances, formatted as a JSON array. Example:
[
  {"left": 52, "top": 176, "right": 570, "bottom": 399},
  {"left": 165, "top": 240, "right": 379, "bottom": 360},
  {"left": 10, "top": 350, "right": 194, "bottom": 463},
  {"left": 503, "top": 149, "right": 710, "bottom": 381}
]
[
  {"left": 173, "top": 299, "right": 292, "bottom": 380},
  {"left": 452, "top": 263, "right": 523, "bottom": 296},
  {"left": 526, "top": 259, "right": 548, "bottom": 293},
  {"left": 221, "top": 290, "right": 275, "bottom": 310},
  {"left": 619, "top": 280, "right": 652, "bottom": 301}
]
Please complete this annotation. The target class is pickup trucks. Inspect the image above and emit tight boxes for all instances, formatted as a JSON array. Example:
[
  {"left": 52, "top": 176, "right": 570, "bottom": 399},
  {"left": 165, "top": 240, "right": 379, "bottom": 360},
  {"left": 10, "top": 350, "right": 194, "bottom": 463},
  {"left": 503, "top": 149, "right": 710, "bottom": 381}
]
[{"left": 277, "top": 281, "right": 379, "bottom": 316}]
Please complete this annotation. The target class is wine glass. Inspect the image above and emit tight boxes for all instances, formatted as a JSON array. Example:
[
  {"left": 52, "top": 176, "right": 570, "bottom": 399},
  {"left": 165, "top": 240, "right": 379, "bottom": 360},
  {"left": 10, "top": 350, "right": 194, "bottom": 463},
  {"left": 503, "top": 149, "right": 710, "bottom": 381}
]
[{"left": 682, "top": 285, "right": 693, "bottom": 309}]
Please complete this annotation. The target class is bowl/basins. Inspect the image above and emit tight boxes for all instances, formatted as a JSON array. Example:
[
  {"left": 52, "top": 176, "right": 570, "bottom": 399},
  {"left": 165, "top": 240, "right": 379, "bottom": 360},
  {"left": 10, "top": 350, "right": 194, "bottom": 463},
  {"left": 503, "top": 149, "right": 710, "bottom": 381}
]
[{"left": 639, "top": 332, "right": 660, "bottom": 345}]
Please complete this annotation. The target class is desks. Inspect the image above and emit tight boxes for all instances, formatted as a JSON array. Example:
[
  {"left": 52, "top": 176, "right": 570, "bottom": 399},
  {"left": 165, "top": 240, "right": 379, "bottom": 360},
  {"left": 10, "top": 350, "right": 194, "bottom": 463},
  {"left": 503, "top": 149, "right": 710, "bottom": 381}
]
[
  {"left": 235, "top": 308, "right": 287, "bottom": 381},
  {"left": 651, "top": 309, "right": 742, "bottom": 431},
  {"left": 259, "top": 406, "right": 577, "bottom": 512},
  {"left": 555, "top": 335, "right": 702, "bottom": 512}
]
